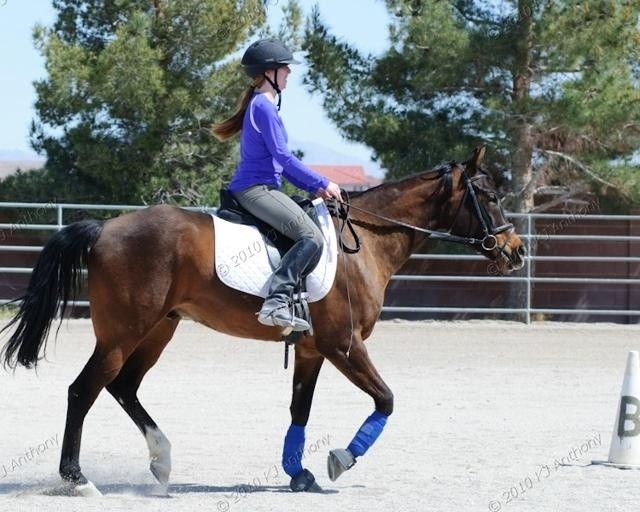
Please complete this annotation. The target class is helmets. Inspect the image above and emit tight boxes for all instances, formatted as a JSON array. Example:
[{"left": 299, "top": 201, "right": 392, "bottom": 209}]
[{"left": 241, "top": 41, "right": 301, "bottom": 78}]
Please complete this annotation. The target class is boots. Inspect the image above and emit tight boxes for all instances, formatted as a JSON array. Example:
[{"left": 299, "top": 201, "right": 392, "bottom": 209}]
[{"left": 257, "top": 236, "right": 323, "bottom": 331}]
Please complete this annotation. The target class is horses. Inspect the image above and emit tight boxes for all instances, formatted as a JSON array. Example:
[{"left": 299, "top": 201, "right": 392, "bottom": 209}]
[{"left": 0, "top": 142, "right": 530, "bottom": 499}]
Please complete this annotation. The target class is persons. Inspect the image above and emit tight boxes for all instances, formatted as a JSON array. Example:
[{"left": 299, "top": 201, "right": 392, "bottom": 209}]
[{"left": 209, "top": 36, "right": 344, "bottom": 332}]
